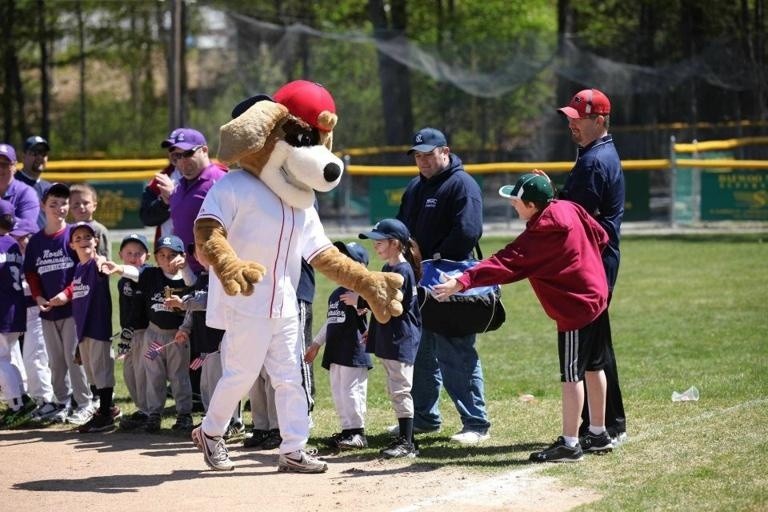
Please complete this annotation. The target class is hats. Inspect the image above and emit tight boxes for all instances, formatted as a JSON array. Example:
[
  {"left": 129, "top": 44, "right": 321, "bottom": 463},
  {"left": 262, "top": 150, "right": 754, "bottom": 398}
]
[
  {"left": 359, "top": 218, "right": 410, "bottom": 247},
  {"left": 333, "top": 241, "right": 369, "bottom": 266},
  {"left": 120, "top": 232, "right": 148, "bottom": 254},
  {"left": 0, "top": 143, "right": 17, "bottom": 163},
  {"left": 154, "top": 235, "right": 185, "bottom": 254},
  {"left": 499, "top": 173, "right": 554, "bottom": 205},
  {"left": 407, "top": 128, "right": 447, "bottom": 156},
  {"left": 556, "top": 88, "right": 611, "bottom": 120},
  {"left": 69, "top": 221, "right": 96, "bottom": 242},
  {"left": 161, "top": 128, "right": 207, "bottom": 152},
  {"left": 24, "top": 136, "right": 50, "bottom": 151},
  {"left": 44, "top": 182, "right": 70, "bottom": 197},
  {"left": 0, "top": 198, "right": 16, "bottom": 217}
]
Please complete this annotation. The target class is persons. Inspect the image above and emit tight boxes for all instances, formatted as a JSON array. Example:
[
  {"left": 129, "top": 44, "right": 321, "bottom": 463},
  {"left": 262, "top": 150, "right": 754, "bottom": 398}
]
[
  {"left": 386, "top": 125, "right": 492, "bottom": 448},
  {"left": 1, "top": 122, "right": 316, "bottom": 451},
  {"left": 304, "top": 236, "right": 372, "bottom": 453},
  {"left": 532, "top": 86, "right": 625, "bottom": 440},
  {"left": 432, "top": 173, "right": 615, "bottom": 464},
  {"left": 338, "top": 218, "right": 425, "bottom": 462}
]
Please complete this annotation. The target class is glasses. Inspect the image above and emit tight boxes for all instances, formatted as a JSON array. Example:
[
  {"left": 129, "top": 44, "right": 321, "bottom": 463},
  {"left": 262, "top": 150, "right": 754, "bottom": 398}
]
[
  {"left": 174, "top": 145, "right": 204, "bottom": 160},
  {"left": 26, "top": 148, "right": 47, "bottom": 157}
]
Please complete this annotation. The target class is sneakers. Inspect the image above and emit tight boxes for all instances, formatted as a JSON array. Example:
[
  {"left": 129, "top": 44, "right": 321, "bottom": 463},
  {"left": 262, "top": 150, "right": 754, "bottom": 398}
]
[
  {"left": 191, "top": 425, "right": 233, "bottom": 471},
  {"left": 530, "top": 418, "right": 627, "bottom": 462},
  {"left": 326, "top": 423, "right": 492, "bottom": 460},
  {"left": 280, "top": 447, "right": 328, "bottom": 474},
  {"left": 0, "top": 392, "right": 282, "bottom": 449}
]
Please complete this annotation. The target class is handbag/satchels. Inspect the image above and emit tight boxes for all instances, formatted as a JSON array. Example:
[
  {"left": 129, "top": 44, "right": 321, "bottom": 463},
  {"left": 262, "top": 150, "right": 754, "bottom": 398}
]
[{"left": 416, "top": 258, "right": 505, "bottom": 338}]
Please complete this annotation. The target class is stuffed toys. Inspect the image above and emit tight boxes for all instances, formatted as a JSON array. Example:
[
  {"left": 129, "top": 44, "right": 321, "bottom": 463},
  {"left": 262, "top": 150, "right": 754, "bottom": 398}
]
[{"left": 192, "top": 82, "right": 407, "bottom": 475}]
